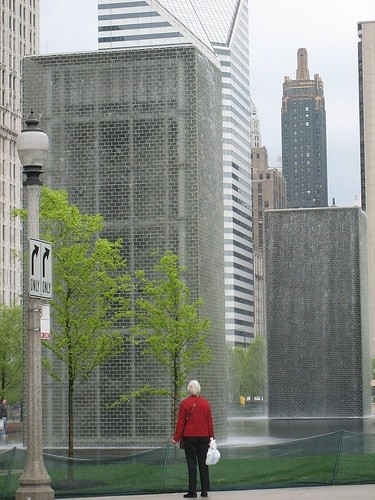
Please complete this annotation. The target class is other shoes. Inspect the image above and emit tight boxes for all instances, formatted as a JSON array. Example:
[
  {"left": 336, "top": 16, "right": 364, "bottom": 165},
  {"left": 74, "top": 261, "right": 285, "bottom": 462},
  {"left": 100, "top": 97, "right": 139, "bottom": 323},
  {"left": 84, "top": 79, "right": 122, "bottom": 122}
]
[
  {"left": 184, "top": 492, "right": 196, "bottom": 498},
  {"left": 201, "top": 491, "right": 207, "bottom": 497}
]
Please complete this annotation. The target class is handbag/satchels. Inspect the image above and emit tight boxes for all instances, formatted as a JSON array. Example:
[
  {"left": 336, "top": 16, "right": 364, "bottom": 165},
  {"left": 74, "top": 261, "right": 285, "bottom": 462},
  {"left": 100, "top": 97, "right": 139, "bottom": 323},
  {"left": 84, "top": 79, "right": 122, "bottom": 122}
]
[
  {"left": 205, "top": 440, "right": 221, "bottom": 465},
  {"left": 180, "top": 434, "right": 184, "bottom": 448}
]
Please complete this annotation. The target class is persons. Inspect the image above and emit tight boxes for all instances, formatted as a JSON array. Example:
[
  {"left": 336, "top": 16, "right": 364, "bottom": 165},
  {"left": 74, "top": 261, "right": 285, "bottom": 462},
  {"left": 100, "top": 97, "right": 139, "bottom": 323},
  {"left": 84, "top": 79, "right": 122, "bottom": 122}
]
[{"left": 171, "top": 380, "right": 215, "bottom": 498}]
